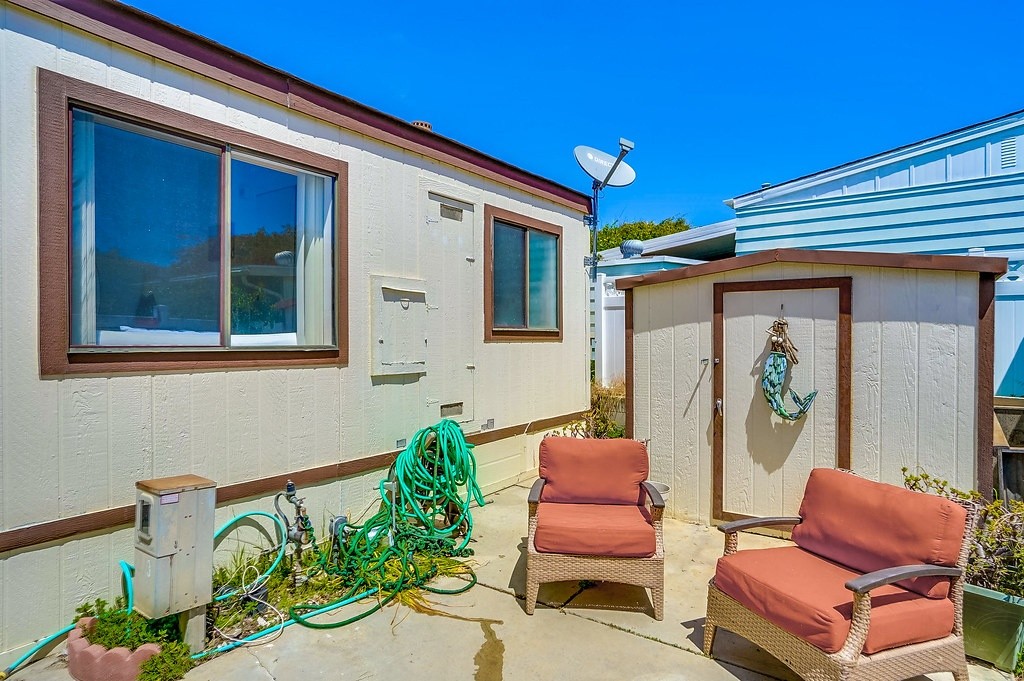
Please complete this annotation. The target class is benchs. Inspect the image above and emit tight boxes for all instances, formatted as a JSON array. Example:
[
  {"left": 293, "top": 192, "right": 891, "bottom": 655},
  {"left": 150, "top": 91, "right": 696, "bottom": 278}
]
[{"left": 703, "top": 466, "right": 980, "bottom": 681}]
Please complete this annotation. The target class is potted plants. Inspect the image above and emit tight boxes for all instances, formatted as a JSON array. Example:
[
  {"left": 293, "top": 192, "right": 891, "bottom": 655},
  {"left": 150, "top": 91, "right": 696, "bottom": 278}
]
[{"left": 904, "top": 468, "right": 1024, "bottom": 674}]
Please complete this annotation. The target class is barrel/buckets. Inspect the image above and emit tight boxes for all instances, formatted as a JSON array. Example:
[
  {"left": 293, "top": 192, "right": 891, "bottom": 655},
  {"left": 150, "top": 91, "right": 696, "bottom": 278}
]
[{"left": 644, "top": 480, "right": 671, "bottom": 517}]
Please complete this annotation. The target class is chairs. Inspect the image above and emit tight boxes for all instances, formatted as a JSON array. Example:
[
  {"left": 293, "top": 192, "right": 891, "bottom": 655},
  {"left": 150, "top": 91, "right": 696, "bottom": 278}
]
[{"left": 525, "top": 436, "right": 667, "bottom": 623}]
